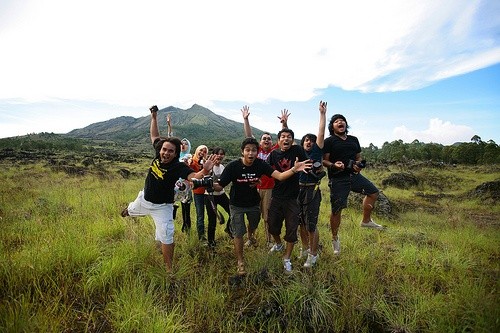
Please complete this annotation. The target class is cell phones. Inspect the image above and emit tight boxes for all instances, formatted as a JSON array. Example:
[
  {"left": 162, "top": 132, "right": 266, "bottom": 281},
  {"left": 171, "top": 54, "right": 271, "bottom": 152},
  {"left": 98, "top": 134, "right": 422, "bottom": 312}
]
[{"left": 150, "top": 106, "right": 158, "bottom": 113}]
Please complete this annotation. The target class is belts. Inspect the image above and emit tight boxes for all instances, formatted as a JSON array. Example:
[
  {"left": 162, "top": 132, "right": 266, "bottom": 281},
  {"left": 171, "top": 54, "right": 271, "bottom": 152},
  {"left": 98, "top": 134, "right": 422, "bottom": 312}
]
[{"left": 299, "top": 182, "right": 316, "bottom": 186}]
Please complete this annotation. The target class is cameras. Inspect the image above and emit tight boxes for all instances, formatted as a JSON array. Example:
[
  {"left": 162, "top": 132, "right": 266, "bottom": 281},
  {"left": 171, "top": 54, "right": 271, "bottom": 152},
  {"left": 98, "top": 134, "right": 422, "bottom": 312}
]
[
  {"left": 309, "top": 162, "right": 326, "bottom": 182},
  {"left": 190, "top": 175, "right": 214, "bottom": 192},
  {"left": 347, "top": 160, "right": 366, "bottom": 169}
]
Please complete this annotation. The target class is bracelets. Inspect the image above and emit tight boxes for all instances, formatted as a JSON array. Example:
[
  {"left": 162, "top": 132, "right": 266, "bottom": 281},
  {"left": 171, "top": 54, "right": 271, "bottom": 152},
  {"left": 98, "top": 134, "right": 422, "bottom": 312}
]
[
  {"left": 332, "top": 164, "right": 335, "bottom": 169},
  {"left": 291, "top": 167, "right": 297, "bottom": 174}
]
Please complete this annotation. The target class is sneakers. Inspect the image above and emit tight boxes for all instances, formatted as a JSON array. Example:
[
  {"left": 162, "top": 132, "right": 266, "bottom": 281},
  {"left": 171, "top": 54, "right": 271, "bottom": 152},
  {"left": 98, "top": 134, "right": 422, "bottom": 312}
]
[
  {"left": 303, "top": 253, "right": 320, "bottom": 268},
  {"left": 360, "top": 219, "right": 384, "bottom": 231},
  {"left": 283, "top": 258, "right": 292, "bottom": 272},
  {"left": 243, "top": 236, "right": 258, "bottom": 246},
  {"left": 297, "top": 248, "right": 311, "bottom": 259},
  {"left": 236, "top": 259, "right": 247, "bottom": 276},
  {"left": 268, "top": 244, "right": 285, "bottom": 257},
  {"left": 331, "top": 234, "right": 341, "bottom": 255}
]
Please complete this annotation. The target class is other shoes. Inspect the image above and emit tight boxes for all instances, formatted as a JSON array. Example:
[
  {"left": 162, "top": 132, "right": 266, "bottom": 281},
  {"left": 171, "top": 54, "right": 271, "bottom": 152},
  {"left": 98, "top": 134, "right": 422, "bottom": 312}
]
[
  {"left": 121, "top": 206, "right": 129, "bottom": 218},
  {"left": 207, "top": 241, "right": 217, "bottom": 256},
  {"left": 182, "top": 228, "right": 192, "bottom": 243},
  {"left": 198, "top": 237, "right": 208, "bottom": 248}
]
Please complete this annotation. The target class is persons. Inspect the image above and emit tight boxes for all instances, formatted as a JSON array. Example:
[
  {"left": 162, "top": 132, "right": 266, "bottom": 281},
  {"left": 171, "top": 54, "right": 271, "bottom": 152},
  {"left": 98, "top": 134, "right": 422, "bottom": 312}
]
[
  {"left": 121, "top": 105, "right": 217, "bottom": 273},
  {"left": 296, "top": 101, "right": 327, "bottom": 268},
  {"left": 240, "top": 105, "right": 291, "bottom": 247},
  {"left": 165, "top": 114, "right": 234, "bottom": 245},
  {"left": 213, "top": 136, "right": 313, "bottom": 275},
  {"left": 264, "top": 129, "right": 306, "bottom": 273},
  {"left": 323, "top": 114, "right": 383, "bottom": 252}
]
